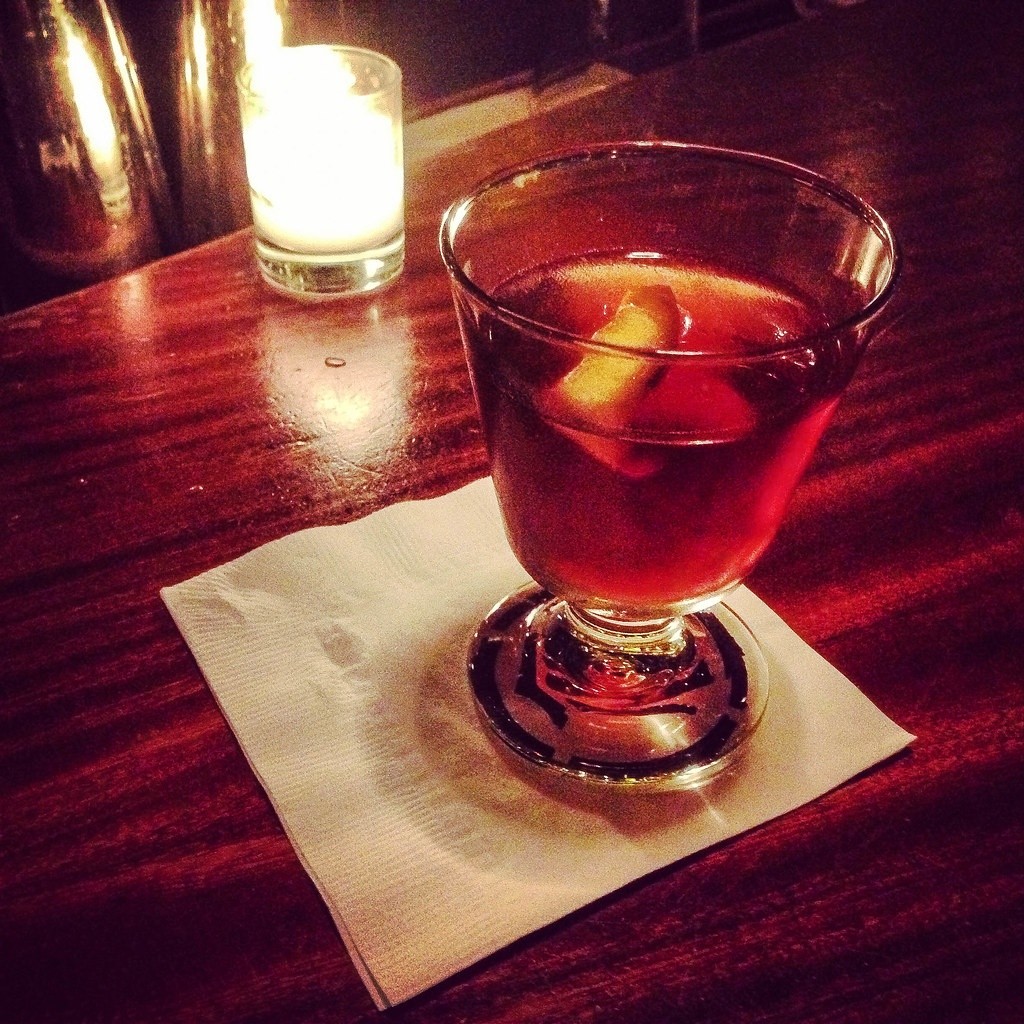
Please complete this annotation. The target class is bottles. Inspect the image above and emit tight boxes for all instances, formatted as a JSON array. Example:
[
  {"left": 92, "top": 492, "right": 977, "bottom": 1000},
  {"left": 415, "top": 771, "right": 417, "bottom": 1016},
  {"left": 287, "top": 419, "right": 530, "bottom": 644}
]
[
  {"left": 0, "top": 0, "right": 173, "bottom": 285},
  {"left": 178, "top": 0, "right": 370, "bottom": 253}
]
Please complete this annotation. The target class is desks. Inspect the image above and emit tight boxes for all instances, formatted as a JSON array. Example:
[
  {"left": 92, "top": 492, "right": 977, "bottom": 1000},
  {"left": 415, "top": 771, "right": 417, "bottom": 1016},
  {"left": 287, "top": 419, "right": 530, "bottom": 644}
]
[{"left": 0, "top": 0, "right": 1024, "bottom": 1024}]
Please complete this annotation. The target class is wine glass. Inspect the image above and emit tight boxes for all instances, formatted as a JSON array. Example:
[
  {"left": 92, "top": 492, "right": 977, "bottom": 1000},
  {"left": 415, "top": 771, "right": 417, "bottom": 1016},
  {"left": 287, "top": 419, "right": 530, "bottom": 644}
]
[{"left": 437, "top": 140, "right": 902, "bottom": 792}]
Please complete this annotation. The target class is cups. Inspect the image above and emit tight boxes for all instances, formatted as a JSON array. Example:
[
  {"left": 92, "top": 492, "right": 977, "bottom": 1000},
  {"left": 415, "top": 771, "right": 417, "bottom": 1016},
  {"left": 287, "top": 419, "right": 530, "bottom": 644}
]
[{"left": 234, "top": 43, "right": 407, "bottom": 303}]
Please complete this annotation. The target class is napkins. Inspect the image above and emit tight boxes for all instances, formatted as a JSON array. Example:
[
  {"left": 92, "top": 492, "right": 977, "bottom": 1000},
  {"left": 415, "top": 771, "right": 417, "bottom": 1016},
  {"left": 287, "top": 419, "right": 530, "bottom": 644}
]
[{"left": 161, "top": 474, "right": 918, "bottom": 1011}]
